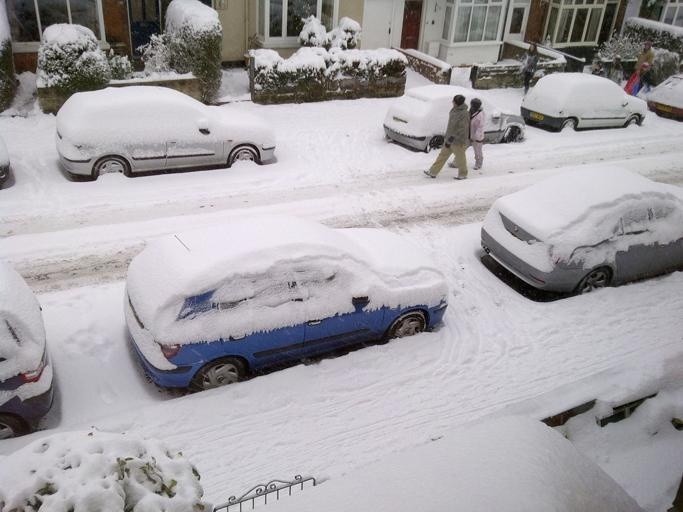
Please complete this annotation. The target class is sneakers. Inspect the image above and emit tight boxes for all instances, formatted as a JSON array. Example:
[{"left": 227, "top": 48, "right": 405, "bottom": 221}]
[
  {"left": 454, "top": 175, "right": 468, "bottom": 180},
  {"left": 448, "top": 162, "right": 457, "bottom": 168},
  {"left": 474, "top": 166, "right": 481, "bottom": 170},
  {"left": 425, "top": 170, "right": 436, "bottom": 178}
]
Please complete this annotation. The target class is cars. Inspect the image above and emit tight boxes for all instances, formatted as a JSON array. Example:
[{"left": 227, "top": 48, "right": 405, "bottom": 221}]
[
  {"left": 480, "top": 164, "right": 683, "bottom": 296},
  {"left": 55, "top": 86, "right": 274, "bottom": 179},
  {"left": 0, "top": 263, "right": 53, "bottom": 440},
  {"left": 648, "top": 74, "right": 683, "bottom": 119},
  {"left": 0, "top": 141, "right": 9, "bottom": 190},
  {"left": 123, "top": 215, "right": 448, "bottom": 393},
  {"left": 383, "top": 84, "right": 525, "bottom": 153}
]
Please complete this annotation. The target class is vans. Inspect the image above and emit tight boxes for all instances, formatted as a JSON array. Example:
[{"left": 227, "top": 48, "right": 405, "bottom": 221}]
[{"left": 521, "top": 73, "right": 647, "bottom": 131}]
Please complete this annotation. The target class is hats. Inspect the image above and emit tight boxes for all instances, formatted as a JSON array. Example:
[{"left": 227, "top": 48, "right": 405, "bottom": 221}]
[
  {"left": 640, "top": 62, "right": 648, "bottom": 68},
  {"left": 644, "top": 41, "right": 652, "bottom": 46}
]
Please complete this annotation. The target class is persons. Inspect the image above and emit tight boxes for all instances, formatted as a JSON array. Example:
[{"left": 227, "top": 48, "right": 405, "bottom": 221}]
[
  {"left": 634, "top": 41, "right": 655, "bottom": 93},
  {"left": 612, "top": 55, "right": 624, "bottom": 85},
  {"left": 515, "top": 42, "right": 539, "bottom": 95},
  {"left": 423, "top": 94, "right": 469, "bottom": 180},
  {"left": 447, "top": 97, "right": 485, "bottom": 171}
]
